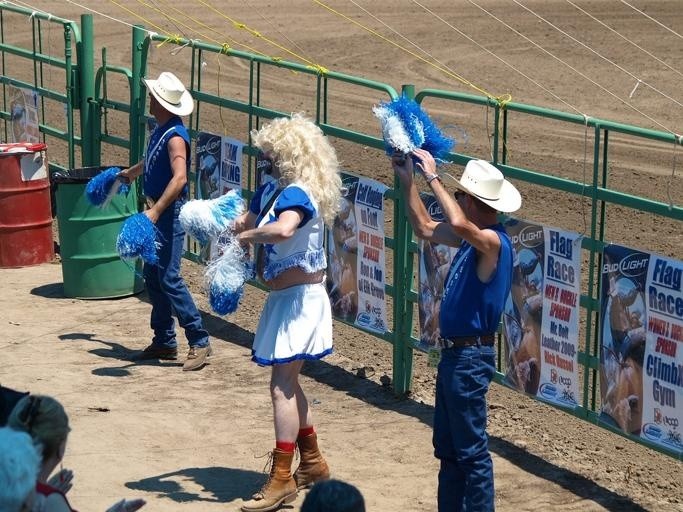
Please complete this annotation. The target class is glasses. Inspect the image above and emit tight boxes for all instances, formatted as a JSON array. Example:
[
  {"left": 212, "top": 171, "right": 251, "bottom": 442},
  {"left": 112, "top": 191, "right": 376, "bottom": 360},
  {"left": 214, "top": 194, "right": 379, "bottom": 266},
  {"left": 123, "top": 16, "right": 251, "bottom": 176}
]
[{"left": 454, "top": 191, "right": 465, "bottom": 200}]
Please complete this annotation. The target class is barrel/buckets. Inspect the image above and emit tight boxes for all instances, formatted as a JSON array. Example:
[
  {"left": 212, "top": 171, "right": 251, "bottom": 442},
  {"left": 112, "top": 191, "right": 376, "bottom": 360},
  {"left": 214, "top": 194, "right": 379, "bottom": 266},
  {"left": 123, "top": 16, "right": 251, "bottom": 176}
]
[{"left": 0, "top": 142, "right": 55, "bottom": 268}]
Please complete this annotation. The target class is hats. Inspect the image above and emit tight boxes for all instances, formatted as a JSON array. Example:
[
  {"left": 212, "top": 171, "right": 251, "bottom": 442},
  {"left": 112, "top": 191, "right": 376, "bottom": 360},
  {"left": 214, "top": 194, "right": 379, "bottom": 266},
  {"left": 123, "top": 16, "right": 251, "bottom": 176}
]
[
  {"left": 441, "top": 159, "right": 522, "bottom": 213},
  {"left": 140, "top": 72, "right": 194, "bottom": 116}
]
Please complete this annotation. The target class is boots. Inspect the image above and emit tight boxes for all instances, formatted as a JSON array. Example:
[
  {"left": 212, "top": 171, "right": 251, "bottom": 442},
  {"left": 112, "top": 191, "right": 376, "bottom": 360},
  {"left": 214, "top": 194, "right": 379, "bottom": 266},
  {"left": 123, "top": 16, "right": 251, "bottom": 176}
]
[
  {"left": 240, "top": 447, "right": 299, "bottom": 511},
  {"left": 293, "top": 433, "right": 330, "bottom": 487}
]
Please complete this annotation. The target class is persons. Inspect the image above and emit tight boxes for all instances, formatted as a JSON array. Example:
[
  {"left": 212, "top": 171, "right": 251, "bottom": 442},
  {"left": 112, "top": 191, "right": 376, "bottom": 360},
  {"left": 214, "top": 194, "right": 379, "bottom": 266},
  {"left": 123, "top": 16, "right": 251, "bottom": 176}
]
[
  {"left": 298, "top": 477, "right": 366, "bottom": 512},
  {"left": 117, "top": 70, "right": 213, "bottom": 372},
  {"left": 230, "top": 112, "right": 347, "bottom": 512},
  {"left": 0, "top": 426, "right": 42, "bottom": 512},
  {"left": 8, "top": 395, "right": 148, "bottom": 512},
  {"left": 391, "top": 147, "right": 525, "bottom": 510}
]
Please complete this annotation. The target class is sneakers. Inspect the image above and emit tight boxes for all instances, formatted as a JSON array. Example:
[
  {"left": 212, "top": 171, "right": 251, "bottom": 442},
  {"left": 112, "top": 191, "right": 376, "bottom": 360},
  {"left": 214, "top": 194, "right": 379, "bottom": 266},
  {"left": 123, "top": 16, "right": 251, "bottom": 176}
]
[
  {"left": 134, "top": 342, "right": 177, "bottom": 360},
  {"left": 182, "top": 344, "right": 214, "bottom": 371}
]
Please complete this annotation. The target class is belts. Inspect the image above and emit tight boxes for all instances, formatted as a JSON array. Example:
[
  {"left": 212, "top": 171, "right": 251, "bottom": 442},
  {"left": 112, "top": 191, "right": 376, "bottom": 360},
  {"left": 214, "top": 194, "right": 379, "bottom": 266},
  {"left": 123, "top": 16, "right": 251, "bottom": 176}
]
[{"left": 438, "top": 335, "right": 495, "bottom": 349}]
